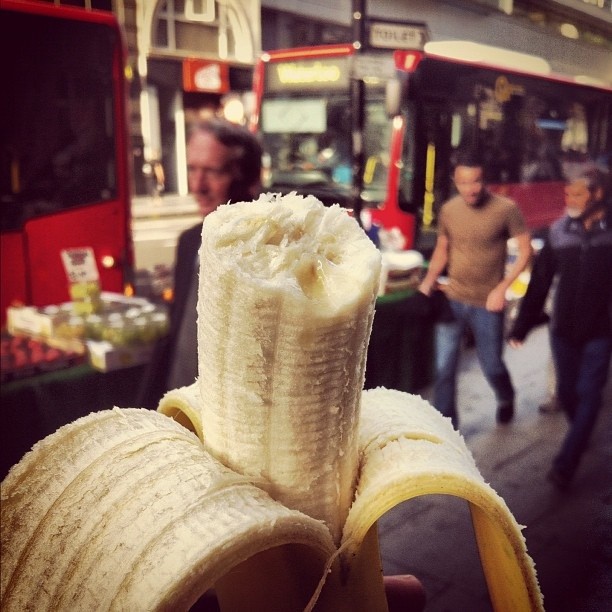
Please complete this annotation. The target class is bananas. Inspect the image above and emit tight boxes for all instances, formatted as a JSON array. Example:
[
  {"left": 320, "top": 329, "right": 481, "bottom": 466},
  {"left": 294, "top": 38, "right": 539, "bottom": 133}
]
[{"left": 1, "top": 189, "right": 548, "bottom": 612}]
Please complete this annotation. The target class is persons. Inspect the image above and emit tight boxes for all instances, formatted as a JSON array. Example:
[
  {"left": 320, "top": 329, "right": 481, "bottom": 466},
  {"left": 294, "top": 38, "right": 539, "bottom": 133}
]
[
  {"left": 418, "top": 151, "right": 531, "bottom": 431},
  {"left": 384, "top": 574, "right": 425, "bottom": 612},
  {"left": 536, "top": 162, "right": 612, "bottom": 414},
  {"left": 504, "top": 167, "right": 612, "bottom": 489},
  {"left": 140, "top": 118, "right": 265, "bottom": 411}
]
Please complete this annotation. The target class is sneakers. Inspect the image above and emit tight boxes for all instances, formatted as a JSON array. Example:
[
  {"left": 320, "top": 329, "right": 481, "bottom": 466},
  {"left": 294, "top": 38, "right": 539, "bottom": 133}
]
[
  {"left": 496, "top": 388, "right": 513, "bottom": 424},
  {"left": 540, "top": 393, "right": 562, "bottom": 415},
  {"left": 549, "top": 462, "right": 574, "bottom": 481}
]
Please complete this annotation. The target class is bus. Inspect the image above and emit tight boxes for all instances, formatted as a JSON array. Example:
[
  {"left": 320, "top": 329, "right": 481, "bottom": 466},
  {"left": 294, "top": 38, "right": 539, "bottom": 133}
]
[
  {"left": 0, "top": 2, "right": 136, "bottom": 317},
  {"left": 246, "top": 45, "right": 610, "bottom": 249}
]
